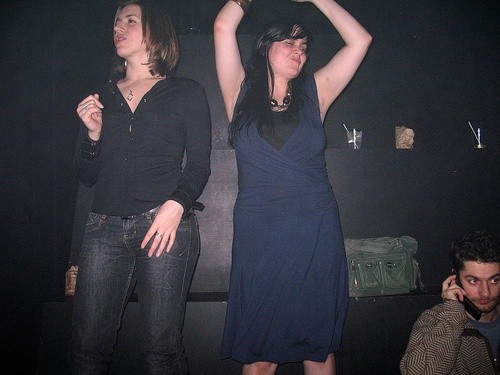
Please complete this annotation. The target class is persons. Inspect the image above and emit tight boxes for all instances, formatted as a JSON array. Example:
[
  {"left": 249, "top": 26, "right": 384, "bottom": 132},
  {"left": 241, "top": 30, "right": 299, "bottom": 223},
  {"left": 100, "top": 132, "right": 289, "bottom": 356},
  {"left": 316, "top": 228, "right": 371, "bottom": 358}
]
[
  {"left": 68, "top": 1, "right": 211, "bottom": 374},
  {"left": 212, "top": 0, "right": 372, "bottom": 375},
  {"left": 398, "top": 225, "right": 499, "bottom": 375}
]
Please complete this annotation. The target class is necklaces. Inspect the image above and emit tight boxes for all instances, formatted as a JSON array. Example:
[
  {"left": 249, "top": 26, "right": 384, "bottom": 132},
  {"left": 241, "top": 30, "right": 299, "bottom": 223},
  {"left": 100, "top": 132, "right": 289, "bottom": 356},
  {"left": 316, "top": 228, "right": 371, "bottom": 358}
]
[
  {"left": 270, "top": 80, "right": 292, "bottom": 110},
  {"left": 122, "top": 76, "right": 162, "bottom": 101}
]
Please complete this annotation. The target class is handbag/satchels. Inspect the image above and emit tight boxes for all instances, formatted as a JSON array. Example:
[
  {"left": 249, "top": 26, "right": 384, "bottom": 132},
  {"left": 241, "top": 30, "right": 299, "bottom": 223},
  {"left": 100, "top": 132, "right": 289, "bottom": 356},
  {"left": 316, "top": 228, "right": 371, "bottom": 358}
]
[{"left": 344, "top": 236, "right": 431, "bottom": 297}]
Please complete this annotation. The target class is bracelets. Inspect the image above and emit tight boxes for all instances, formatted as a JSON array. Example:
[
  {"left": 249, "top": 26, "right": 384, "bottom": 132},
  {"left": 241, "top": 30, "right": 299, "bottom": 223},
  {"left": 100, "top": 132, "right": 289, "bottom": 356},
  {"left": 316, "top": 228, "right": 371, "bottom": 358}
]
[{"left": 232, "top": 0, "right": 252, "bottom": 14}]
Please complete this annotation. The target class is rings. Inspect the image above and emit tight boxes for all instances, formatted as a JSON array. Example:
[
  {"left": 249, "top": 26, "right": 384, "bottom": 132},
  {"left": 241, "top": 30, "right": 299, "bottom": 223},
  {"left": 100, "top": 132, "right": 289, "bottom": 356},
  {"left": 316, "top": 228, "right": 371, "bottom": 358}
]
[
  {"left": 156, "top": 233, "right": 164, "bottom": 238},
  {"left": 84, "top": 106, "right": 88, "bottom": 111}
]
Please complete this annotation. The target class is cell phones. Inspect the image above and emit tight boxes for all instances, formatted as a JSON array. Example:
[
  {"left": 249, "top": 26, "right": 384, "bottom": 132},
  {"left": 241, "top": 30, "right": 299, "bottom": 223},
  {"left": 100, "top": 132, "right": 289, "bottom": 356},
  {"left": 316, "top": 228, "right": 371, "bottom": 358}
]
[{"left": 453, "top": 270, "right": 482, "bottom": 322}]
